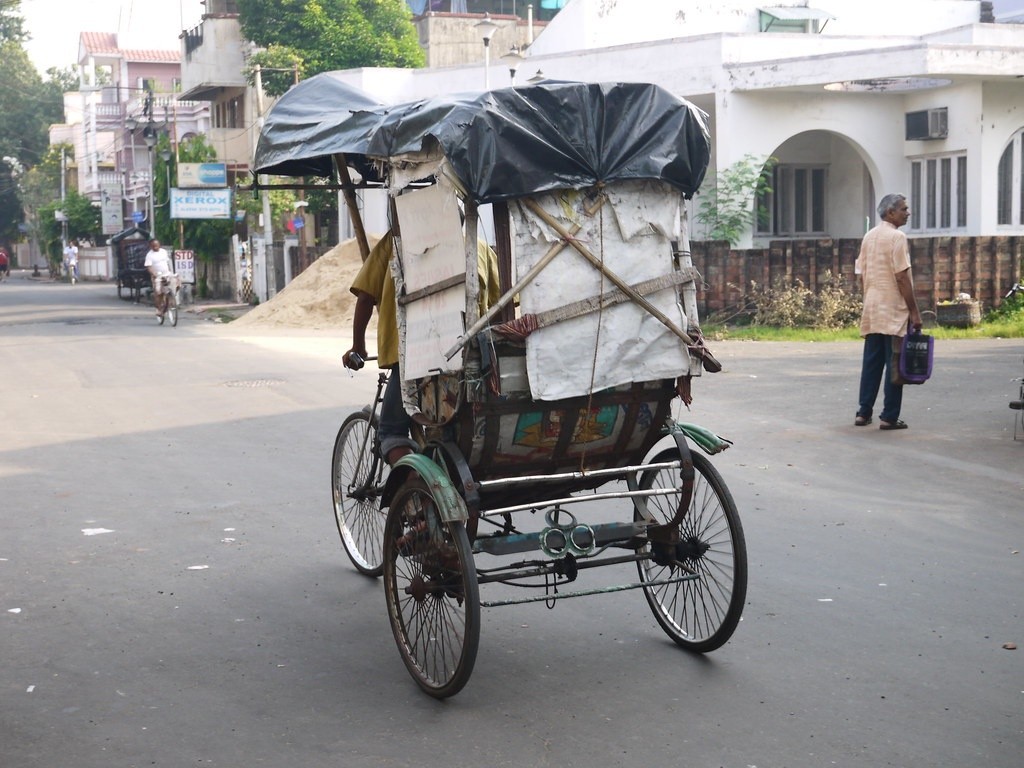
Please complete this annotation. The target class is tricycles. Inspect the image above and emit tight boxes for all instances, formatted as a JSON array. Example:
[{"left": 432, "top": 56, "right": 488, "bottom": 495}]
[
  {"left": 247, "top": 64, "right": 745, "bottom": 699},
  {"left": 111, "top": 228, "right": 156, "bottom": 304}
]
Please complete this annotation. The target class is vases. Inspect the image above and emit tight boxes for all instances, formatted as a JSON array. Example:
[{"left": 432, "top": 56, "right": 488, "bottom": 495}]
[{"left": 936, "top": 297, "right": 981, "bottom": 329}]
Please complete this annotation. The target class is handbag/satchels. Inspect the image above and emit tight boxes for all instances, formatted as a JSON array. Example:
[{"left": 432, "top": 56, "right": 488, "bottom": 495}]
[
  {"left": 891, "top": 335, "right": 925, "bottom": 385},
  {"left": 900, "top": 315, "right": 934, "bottom": 381}
]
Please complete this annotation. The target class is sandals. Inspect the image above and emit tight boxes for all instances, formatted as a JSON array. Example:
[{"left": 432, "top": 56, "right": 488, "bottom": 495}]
[
  {"left": 855, "top": 412, "right": 873, "bottom": 425},
  {"left": 399, "top": 529, "right": 442, "bottom": 546},
  {"left": 879, "top": 416, "right": 908, "bottom": 429}
]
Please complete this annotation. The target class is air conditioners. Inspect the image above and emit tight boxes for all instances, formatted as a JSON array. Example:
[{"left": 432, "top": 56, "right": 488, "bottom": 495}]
[{"left": 905, "top": 107, "right": 948, "bottom": 141}]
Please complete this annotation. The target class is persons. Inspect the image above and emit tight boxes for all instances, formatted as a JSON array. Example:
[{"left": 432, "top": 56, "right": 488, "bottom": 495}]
[
  {"left": 341, "top": 194, "right": 521, "bottom": 558},
  {"left": 0, "top": 246, "right": 10, "bottom": 282},
  {"left": 143, "top": 238, "right": 180, "bottom": 315},
  {"left": 62, "top": 240, "right": 80, "bottom": 281},
  {"left": 854, "top": 191, "right": 924, "bottom": 432}
]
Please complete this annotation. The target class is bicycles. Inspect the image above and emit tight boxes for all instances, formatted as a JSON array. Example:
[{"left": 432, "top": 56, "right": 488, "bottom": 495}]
[
  {"left": 155, "top": 273, "right": 180, "bottom": 327},
  {"left": 63, "top": 257, "right": 79, "bottom": 285}
]
